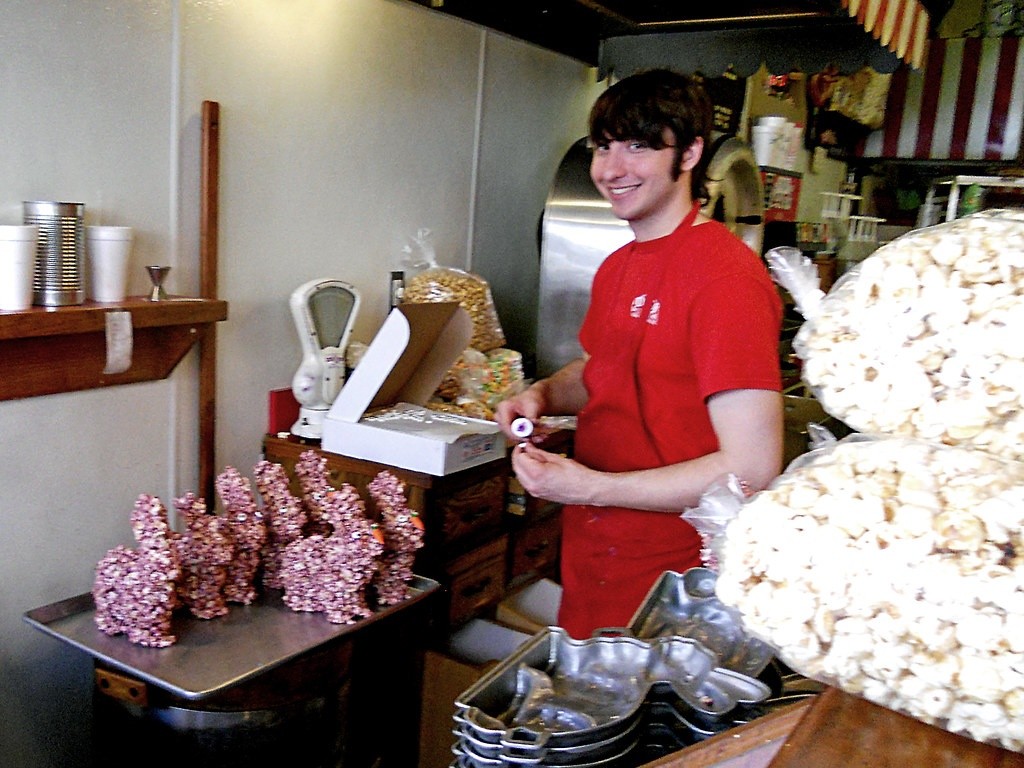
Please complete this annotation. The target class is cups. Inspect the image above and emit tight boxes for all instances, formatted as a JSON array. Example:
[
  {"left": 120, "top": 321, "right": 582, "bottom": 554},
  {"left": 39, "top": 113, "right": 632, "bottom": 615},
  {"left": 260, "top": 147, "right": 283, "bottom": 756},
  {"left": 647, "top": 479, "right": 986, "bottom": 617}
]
[
  {"left": 22, "top": 201, "right": 87, "bottom": 305},
  {"left": 84, "top": 226, "right": 134, "bottom": 303},
  {"left": 0, "top": 225, "right": 38, "bottom": 310},
  {"left": 752, "top": 117, "right": 802, "bottom": 170}
]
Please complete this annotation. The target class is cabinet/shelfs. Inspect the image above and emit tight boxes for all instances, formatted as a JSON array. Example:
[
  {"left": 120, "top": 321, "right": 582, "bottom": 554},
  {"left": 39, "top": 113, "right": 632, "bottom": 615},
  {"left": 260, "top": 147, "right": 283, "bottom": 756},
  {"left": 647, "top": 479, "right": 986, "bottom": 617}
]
[{"left": 820, "top": 192, "right": 887, "bottom": 243}]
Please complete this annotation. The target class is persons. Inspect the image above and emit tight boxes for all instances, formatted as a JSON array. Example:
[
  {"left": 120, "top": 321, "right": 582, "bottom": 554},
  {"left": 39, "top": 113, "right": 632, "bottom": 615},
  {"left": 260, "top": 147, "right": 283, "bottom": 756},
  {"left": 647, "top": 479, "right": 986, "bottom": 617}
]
[{"left": 492, "top": 69, "right": 783, "bottom": 638}]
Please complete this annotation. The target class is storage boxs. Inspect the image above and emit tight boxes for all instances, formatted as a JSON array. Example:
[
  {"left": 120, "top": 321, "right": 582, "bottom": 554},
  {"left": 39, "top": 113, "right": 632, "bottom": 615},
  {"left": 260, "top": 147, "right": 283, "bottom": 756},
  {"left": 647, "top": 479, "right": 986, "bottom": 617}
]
[
  {"left": 418, "top": 619, "right": 534, "bottom": 768},
  {"left": 496, "top": 576, "right": 563, "bottom": 634},
  {"left": 442, "top": 532, "right": 508, "bottom": 620},
  {"left": 261, "top": 435, "right": 511, "bottom": 551},
  {"left": 507, "top": 426, "right": 576, "bottom": 517},
  {"left": 509, "top": 517, "right": 563, "bottom": 589},
  {"left": 320, "top": 300, "right": 507, "bottom": 477}
]
